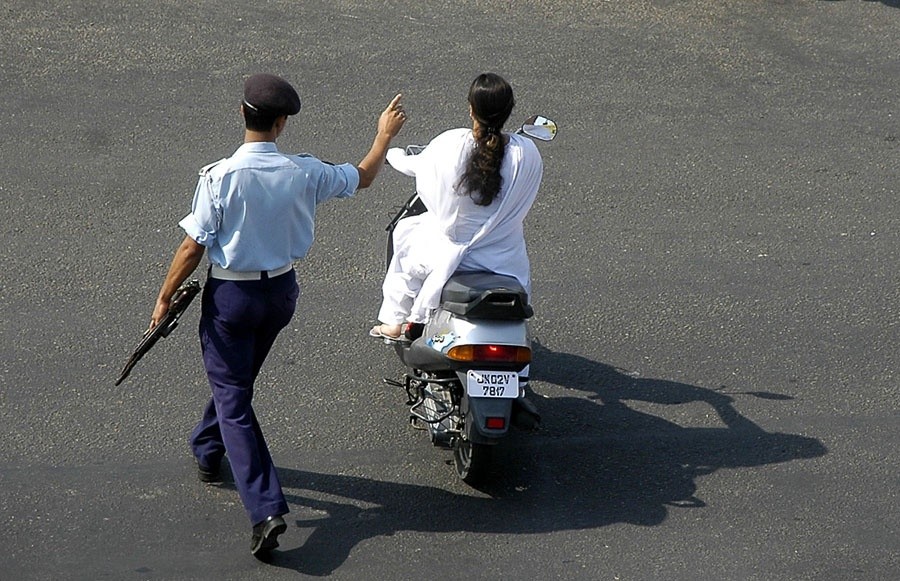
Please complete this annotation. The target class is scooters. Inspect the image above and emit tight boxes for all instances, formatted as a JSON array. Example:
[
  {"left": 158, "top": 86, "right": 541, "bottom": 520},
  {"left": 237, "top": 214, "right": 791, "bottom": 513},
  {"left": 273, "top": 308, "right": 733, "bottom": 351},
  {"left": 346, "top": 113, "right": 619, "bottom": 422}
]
[{"left": 382, "top": 115, "right": 559, "bottom": 485}]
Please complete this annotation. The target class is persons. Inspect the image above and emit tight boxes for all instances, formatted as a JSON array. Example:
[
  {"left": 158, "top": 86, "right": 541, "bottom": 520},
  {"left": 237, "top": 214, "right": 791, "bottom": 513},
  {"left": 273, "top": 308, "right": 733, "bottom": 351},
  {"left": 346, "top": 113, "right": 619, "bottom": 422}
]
[
  {"left": 147, "top": 73, "right": 408, "bottom": 555},
  {"left": 370, "top": 70, "right": 544, "bottom": 347}
]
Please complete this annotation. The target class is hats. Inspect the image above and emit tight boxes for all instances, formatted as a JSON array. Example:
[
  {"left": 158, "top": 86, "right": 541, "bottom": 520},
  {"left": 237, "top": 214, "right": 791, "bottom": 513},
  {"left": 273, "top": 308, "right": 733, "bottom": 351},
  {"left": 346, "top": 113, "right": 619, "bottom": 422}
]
[{"left": 242, "top": 73, "right": 302, "bottom": 117}]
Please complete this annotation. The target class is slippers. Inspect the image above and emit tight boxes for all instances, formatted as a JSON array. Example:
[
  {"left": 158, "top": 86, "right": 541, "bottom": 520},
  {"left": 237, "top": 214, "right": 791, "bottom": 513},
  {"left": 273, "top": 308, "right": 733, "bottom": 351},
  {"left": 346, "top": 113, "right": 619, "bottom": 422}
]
[{"left": 370, "top": 324, "right": 401, "bottom": 341}]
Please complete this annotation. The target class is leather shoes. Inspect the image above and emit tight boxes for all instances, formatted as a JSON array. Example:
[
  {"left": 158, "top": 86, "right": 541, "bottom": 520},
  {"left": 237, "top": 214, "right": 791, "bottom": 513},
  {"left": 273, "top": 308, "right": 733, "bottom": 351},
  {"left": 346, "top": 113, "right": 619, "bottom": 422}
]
[
  {"left": 198, "top": 455, "right": 229, "bottom": 482},
  {"left": 250, "top": 514, "right": 287, "bottom": 556}
]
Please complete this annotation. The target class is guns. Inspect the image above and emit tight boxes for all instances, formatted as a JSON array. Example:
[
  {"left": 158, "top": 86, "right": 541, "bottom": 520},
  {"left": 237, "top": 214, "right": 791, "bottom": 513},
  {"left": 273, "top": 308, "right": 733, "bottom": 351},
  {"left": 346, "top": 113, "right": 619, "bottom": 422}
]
[{"left": 114, "top": 278, "right": 203, "bottom": 386}]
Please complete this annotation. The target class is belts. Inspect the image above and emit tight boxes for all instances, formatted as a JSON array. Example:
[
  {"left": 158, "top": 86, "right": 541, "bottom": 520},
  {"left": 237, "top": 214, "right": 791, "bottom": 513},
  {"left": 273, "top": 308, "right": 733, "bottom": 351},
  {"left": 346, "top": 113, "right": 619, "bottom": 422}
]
[{"left": 210, "top": 264, "right": 293, "bottom": 281}]
